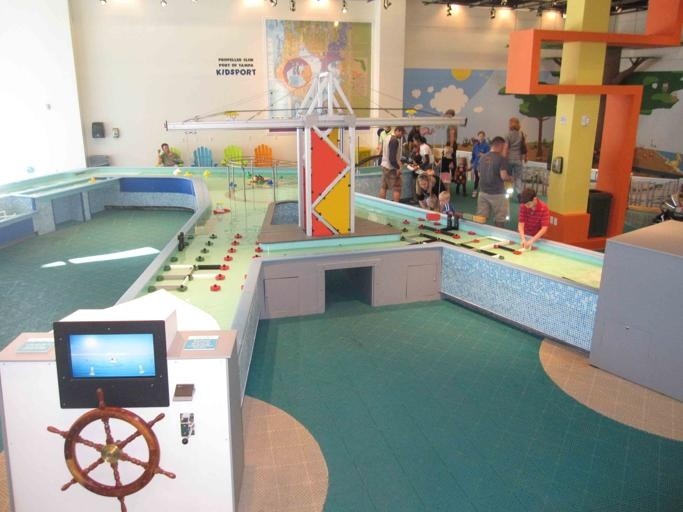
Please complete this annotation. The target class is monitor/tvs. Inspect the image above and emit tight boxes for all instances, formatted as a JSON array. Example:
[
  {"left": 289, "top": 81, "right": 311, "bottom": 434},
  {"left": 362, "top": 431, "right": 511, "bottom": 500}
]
[{"left": 68, "top": 332, "right": 157, "bottom": 379}]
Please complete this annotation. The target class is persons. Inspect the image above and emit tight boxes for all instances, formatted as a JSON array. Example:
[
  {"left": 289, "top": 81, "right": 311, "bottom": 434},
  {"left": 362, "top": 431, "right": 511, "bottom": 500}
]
[
  {"left": 653, "top": 186, "right": 683, "bottom": 223},
  {"left": 375, "top": 110, "right": 552, "bottom": 249},
  {"left": 157, "top": 143, "right": 179, "bottom": 166}
]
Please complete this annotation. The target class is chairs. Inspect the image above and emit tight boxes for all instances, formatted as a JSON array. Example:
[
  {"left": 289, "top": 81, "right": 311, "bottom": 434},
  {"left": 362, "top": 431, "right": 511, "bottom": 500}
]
[
  {"left": 190, "top": 146, "right": 218, "bottom": 167},
  {"left": 255, "top": 143, "right": 273, "bottom": 167},
  {"left": 220, "top": 145, "right": 248, "bottom": 167},
  {"left": 155, "top": 146, "right": 184, "bottom": 167}
]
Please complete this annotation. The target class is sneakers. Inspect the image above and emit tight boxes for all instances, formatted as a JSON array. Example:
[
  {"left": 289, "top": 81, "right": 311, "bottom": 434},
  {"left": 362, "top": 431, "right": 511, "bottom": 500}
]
[
  {"left": 455, "top": 191, "right": 468, "bottom": 196},
  {"left": 471, "top": 190, "right": 477, "bottom": 198}
]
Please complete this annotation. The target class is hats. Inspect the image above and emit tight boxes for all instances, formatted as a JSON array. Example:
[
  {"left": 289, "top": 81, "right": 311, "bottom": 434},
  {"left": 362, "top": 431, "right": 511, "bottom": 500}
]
[{"left": 458, "top": 158, "right": 466, "bottom": 168}]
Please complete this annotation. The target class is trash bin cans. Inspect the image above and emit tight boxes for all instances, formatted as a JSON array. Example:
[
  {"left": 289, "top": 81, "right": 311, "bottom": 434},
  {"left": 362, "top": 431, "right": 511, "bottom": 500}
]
[
  {"left": 587, "top": 190, "right": 613, "bottom": 237},
  {"left": 87, "top": 155, "right": 109, "bottom": 167}
]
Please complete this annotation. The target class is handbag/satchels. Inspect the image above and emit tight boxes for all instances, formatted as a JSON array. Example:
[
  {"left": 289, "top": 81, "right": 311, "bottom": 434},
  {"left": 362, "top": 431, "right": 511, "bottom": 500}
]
[{"left": 519, "top": 131, "right": 527, "bottom": 155}]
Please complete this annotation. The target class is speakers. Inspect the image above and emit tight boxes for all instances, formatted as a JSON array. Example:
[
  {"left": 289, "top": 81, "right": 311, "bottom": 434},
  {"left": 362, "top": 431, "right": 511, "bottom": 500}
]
[{"left": 91, "top": 122, "right": 104, "bottom": 138}]
